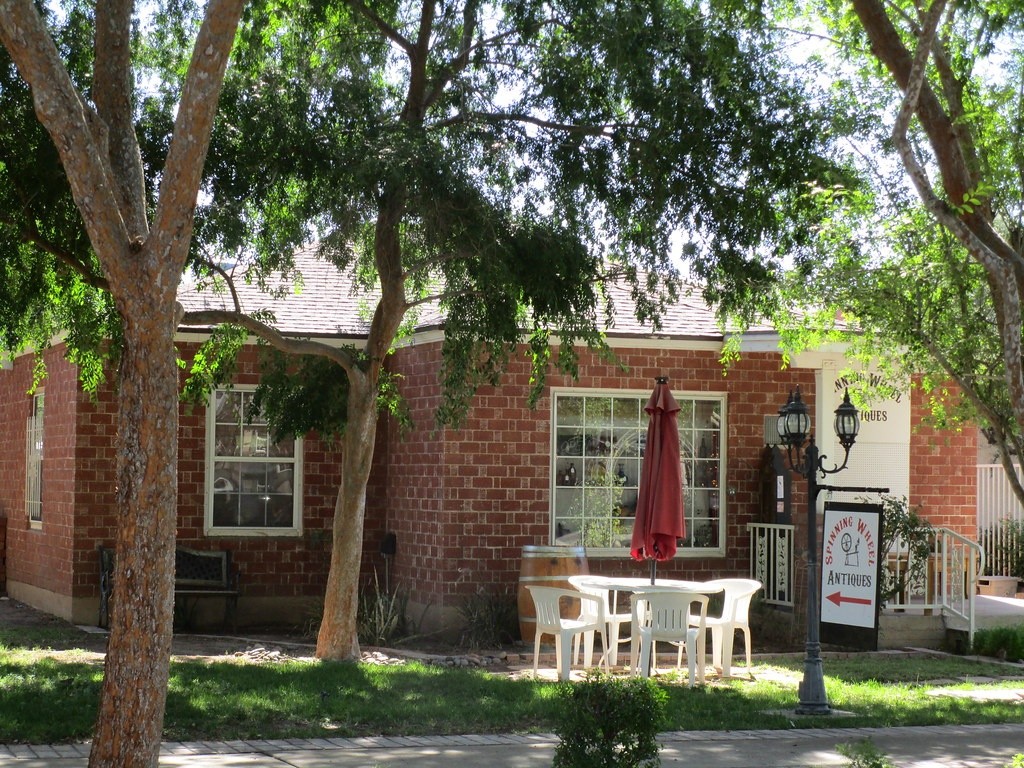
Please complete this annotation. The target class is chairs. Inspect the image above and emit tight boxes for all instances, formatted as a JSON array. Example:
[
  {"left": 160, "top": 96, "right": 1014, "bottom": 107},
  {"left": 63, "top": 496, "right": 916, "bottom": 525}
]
[
  {"left": 525, "top": 585, "right": 610, "bottom": 683},
  {"left": 676, "top": 578, "right": 763, "bottom": 679},
  {"left": 629, "top": 592, "right": 708, "bottom": 689},
  {"left": 569, "top": 574, "right": 657, "bottom": 671}
]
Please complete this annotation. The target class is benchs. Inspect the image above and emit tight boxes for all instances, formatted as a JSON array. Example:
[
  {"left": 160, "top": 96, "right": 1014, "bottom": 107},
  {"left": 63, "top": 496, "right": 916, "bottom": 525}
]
[{"left": 97, "top": 542, "right": 239, "bottom": 633}]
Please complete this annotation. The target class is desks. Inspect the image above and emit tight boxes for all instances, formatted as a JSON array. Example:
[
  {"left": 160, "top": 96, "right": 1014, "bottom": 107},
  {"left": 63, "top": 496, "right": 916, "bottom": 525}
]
[{"left": 581, "top": 579, "right": 723, "bottom": 679}]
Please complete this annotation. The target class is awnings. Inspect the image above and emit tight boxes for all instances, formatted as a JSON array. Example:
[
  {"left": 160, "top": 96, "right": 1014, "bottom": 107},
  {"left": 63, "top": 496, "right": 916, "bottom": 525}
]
[{"left": 630, "top": 374, "right": 685, "bottom": 585}]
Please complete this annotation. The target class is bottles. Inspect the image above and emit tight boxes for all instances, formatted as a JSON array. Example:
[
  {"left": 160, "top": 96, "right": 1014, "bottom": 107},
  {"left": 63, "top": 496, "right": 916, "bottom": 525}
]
[
  {"left": 557, "top": 462, "right": 576, "bottom": 486},
  {"left": 615, "top": 463, "right": 628, "bottom": 486},
  {"left": 700, "top": 438, "right": 707, "bottom": 458}
]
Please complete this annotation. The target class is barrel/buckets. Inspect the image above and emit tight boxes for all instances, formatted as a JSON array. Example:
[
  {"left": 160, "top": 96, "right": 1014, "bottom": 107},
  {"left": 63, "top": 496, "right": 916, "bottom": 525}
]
[{"left": 517, "top": 544, "right": 590, "bottom": 652}]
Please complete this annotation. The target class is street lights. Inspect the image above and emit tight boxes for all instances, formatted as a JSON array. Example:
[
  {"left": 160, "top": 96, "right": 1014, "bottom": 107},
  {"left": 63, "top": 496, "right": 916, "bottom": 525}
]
[{"left": 775, "top": 382, "right": 862, "bottom": 711}]
